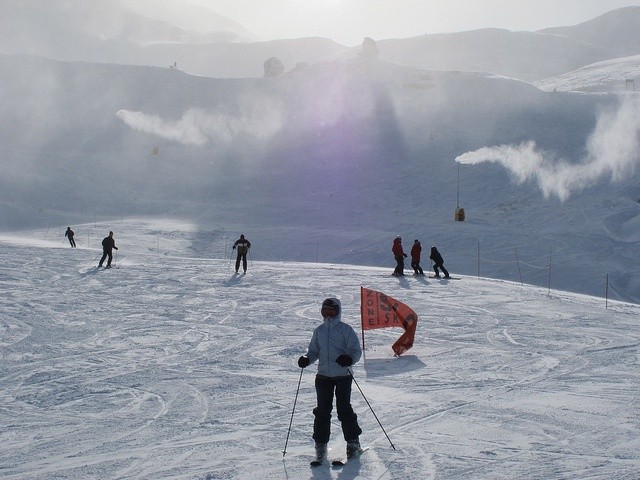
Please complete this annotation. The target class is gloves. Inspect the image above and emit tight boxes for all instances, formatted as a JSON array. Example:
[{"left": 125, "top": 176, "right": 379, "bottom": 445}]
[
  {"left": 298, "top": 356, "right": 310, "bottom": 368},
  {"left": 337, "top": 355, "right": 353, "bottom": 367}
]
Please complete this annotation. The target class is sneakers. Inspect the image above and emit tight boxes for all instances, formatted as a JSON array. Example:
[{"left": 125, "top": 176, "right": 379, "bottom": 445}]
[
  {"left": 346, "top": 437, "right": 361, "bottom": 457},
  {"left": 314, "top": 442, "right": 327, "bottom": 460}
]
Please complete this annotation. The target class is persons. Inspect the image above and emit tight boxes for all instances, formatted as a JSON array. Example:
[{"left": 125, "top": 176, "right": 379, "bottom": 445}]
[
  {"left": 98, "top": 231, "right": 118, "bottom": 269},
  {"left": 232, "top": 235, "right": 250, "bottom": 273},
  {"left": 65, "top": 226, "right": 76, "bottom": 247},
  {"left": 409, "top": 240, "right": 423, "bottom": 275},
  {"left": 429, "top": 247, "right": 450, "bottom": 277},
  {"left": 298, "top": 297, "right": 362, "bottom": 462},
  {"left": 392, "top": 234, "right": 407, "bottom": 276}
]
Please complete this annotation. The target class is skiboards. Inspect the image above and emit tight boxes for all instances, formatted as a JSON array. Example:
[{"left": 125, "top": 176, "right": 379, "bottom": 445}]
[
  {"left": 429, "top": 276, "right": 464, "bottom": 280},
  {"left": 310, "top": 447, "right": 369, "bottom": 466},
  {"left": 372, "top": 273, "right": 405, "bottom": 278},
  {"left": 404, "top": 272, "right": 434, "bottom": 277}
]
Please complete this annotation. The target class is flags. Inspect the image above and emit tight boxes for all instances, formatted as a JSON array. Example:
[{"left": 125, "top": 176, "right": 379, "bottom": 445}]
[{"left": 362, "top": 287, "right": 418, "bottom": 357}]
